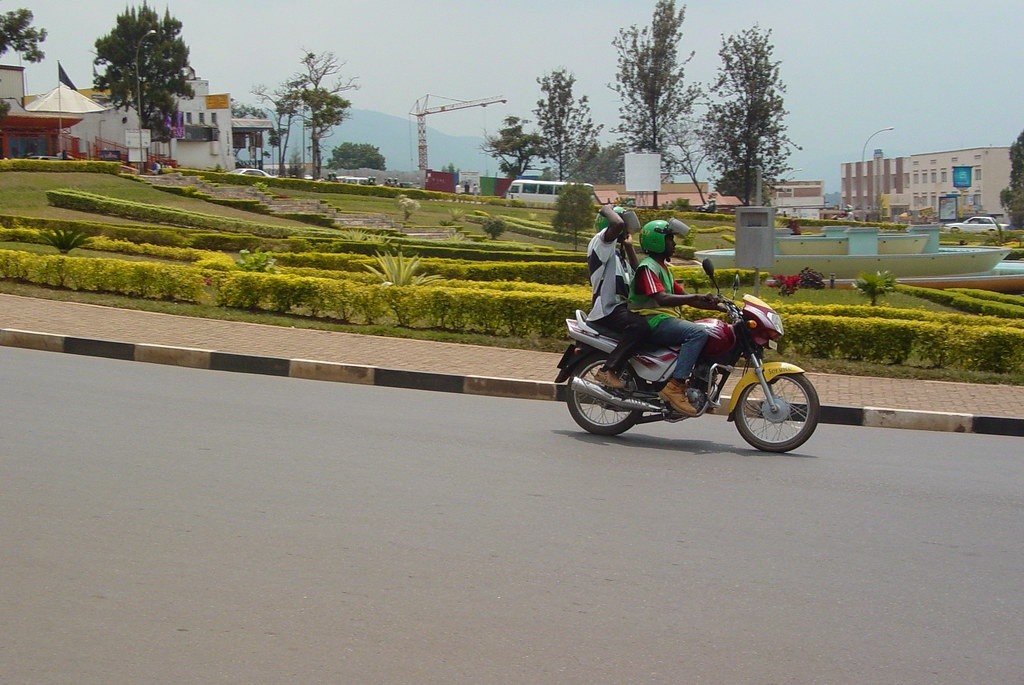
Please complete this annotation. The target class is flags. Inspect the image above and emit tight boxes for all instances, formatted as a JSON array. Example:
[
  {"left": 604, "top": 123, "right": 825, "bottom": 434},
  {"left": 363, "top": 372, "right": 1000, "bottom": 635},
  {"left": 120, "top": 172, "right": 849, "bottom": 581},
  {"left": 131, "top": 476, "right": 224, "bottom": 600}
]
[{"left": 59, "top": 63, "right": 77, "bottom": 91}]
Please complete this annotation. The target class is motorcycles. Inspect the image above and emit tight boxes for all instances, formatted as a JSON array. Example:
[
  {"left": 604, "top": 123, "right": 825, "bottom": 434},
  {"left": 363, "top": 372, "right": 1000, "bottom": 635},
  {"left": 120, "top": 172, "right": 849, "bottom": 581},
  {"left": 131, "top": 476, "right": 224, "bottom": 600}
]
[{"left": 554, "top": 262, "right": 820, "bottom": 452}]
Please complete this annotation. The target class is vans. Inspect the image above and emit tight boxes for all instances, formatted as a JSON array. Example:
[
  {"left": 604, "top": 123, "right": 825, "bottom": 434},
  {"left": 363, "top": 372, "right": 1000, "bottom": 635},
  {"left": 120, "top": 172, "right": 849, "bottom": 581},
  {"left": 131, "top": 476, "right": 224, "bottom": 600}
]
[{"left": 27, "top": 156, "right": 57, "bottom": 160}]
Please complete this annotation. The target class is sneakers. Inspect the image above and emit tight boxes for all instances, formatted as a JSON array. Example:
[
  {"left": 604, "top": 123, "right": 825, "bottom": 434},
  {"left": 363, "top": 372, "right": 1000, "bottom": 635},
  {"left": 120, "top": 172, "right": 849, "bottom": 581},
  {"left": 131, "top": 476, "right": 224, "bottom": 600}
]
[
  {"left": 658, "top": 378, "right": 697, "bottom": 416},
  {"left": 594, "top": 369, "right": 624, "bottom": 388}
]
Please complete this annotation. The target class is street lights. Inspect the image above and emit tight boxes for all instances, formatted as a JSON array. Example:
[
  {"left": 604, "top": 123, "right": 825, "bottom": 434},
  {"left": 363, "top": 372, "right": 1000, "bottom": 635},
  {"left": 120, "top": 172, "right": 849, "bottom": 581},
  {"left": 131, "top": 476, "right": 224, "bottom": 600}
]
[
  {"left": 860, "top": 127, "right": 895, "bottom": 223},
  {"left": 134, "top": 30, "right": 156, "bottom": 175}
]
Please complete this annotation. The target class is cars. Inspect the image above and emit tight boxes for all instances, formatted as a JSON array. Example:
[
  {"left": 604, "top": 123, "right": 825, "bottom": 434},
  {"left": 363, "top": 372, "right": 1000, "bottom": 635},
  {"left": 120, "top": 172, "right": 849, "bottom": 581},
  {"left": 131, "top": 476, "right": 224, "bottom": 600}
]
[
  {"left": 944, "top": 216, "right": 1010, "bottom": 233},
  {"left": 227, "top": 168, "right": 274, "bottom": 178},
  {"left": 303, "top": 174, "right": 371, "bottom": 185}
]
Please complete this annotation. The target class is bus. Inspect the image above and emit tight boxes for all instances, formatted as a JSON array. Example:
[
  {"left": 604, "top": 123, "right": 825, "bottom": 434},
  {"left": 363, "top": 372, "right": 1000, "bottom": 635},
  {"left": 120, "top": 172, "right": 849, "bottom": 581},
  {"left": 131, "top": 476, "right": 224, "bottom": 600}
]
[{"left": 502, "top": 179, "right": 595, "bottom": 206}]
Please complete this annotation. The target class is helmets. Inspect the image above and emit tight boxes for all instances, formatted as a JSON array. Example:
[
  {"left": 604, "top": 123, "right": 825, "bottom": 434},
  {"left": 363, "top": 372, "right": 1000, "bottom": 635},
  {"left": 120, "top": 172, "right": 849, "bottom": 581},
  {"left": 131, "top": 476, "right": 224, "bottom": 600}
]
[
  {"left": 594, "top": 206, "right": 640, "bottom": 242},
  {"left": 639, "top": 217, "right": 691, "bottom": 254}
]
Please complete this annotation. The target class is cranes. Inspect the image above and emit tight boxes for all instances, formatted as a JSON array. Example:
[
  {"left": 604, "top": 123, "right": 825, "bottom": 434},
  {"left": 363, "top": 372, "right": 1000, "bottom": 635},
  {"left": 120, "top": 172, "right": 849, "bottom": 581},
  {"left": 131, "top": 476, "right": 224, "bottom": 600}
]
[{"left": 407, "top": 91, "right": 509, "bottom": 182}]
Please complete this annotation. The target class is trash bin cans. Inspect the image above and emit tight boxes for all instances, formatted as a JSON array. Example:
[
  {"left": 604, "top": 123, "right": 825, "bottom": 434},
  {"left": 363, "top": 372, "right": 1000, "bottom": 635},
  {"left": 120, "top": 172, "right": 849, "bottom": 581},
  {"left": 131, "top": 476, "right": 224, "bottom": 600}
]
[{"left": 734, "top": 205, "right": 776, "bottom": 271}]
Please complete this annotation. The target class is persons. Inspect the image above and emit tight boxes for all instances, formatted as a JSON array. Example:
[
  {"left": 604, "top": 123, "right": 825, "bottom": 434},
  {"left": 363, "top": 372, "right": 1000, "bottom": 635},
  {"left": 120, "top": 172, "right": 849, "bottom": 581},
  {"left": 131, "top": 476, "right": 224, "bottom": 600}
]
[
  {"left": 328, "top": 172, "right": 478, "bottom": 196},
  {"left": 837, "top": 204, "right": 854, "bottom": 220},
  {"left": 614, "top": 197, "right": 669, "bottom": 210},
  {"left": 586, "top": 204, "right": 728, "bottom": 415}
]
